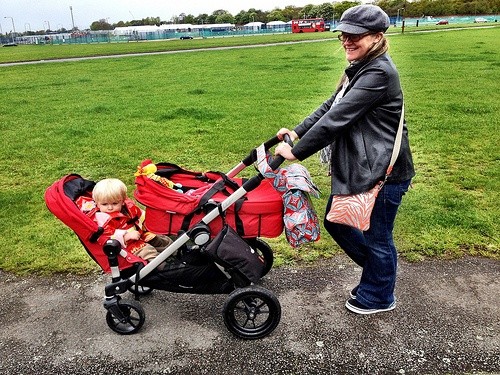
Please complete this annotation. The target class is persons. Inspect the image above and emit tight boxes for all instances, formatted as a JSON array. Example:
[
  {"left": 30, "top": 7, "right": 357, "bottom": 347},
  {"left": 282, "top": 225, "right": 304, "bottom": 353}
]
[
  {"left": 274, "top": 4, "right": 416, "bottom": 314},
  {"left": 90, "top": 177, "right": 186, "bottom": 272}
]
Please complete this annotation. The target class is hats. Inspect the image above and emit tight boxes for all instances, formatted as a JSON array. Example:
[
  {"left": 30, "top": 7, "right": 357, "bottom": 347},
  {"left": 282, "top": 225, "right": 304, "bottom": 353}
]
[{"left": 331, "top": 4, "right": 390, "bottom": 34}]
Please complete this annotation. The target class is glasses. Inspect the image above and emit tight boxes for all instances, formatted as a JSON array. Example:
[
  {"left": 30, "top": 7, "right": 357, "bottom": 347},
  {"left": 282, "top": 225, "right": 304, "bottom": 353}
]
[{"left": 338, "top": 33, "right": 370, "bottom": 43}]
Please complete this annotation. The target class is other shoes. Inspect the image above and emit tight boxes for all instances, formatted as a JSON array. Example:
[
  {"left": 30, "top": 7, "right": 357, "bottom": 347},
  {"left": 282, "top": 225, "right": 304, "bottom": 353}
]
[
  {"left": 163, "top": 263, "right": 178, "bottom": 270},
  {"left": 346, "top": 284, "right": 397, "bottom": 314}
]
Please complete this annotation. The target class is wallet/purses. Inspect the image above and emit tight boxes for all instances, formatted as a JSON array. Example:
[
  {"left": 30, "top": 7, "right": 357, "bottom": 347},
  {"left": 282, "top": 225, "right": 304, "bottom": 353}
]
[{"left": 205, "top": 203, "right": 264, "bottom": 289}]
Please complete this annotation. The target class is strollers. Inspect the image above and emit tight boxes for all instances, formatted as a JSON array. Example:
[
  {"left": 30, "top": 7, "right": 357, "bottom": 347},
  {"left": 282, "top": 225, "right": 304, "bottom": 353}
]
[{"left": 42, "top": 129, "right": 297, "bottom": 340}]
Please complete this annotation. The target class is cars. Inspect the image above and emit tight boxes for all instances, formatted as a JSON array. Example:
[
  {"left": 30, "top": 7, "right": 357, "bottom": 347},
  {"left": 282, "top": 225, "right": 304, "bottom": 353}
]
[
  {"left": 435, "top": 20, "right": 449, "bottom": 26},
  {"left": 180, "top": 35, "right": 193, "bottom": 40},
  {"left": 2, "top": 42, "right": 18, "bottom": 47},
  {"left": 474, "top": 17, "right": 488, "bottom": 24}
]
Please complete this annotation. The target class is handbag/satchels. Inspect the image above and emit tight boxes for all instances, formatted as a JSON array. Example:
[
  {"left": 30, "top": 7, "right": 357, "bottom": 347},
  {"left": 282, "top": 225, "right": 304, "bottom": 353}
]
[
  {"left": 326, "top": 180, "right": 383, "bottom": 231},
  {"left": 256, "top": 143, "right": 323, "bottom": 249}
]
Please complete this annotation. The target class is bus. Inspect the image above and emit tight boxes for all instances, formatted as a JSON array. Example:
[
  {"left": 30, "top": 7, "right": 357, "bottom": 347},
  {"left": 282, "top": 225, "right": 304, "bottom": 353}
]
[{"left": 292, "top": 18, "right": 325, "bottom": 34}]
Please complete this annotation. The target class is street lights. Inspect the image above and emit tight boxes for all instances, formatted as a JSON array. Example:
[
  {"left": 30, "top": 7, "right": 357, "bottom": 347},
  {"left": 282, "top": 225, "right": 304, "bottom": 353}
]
[
  {"left": 44, "top": 20, "right": 51, "bottom": 31},
  {"left": 3, "top": 16, "right": 15, "bottom": 34}
]
